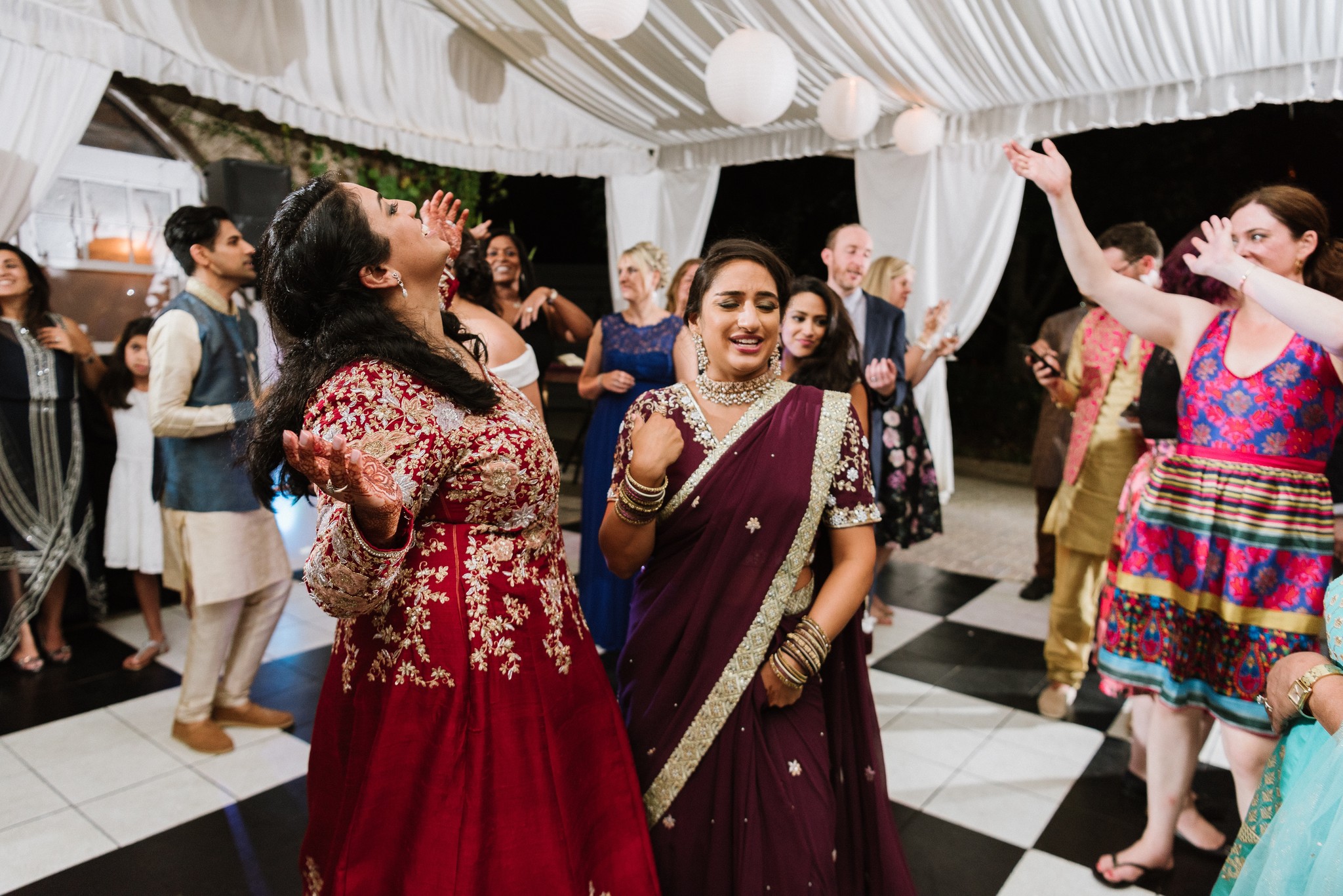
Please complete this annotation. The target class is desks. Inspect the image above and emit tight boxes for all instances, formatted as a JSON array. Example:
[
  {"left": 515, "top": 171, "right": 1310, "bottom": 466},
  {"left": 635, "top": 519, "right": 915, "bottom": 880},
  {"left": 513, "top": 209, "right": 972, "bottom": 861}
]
[{"left": 540, "top": 370, "right": 597, "bottom": 482}]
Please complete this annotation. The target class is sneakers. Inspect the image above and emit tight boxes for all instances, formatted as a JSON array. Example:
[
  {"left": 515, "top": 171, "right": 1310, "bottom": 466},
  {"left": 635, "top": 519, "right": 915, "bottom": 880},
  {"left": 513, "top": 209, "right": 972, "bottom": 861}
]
[{"left": 1036, "top": 681, "right": 1077, "bottom": 716}]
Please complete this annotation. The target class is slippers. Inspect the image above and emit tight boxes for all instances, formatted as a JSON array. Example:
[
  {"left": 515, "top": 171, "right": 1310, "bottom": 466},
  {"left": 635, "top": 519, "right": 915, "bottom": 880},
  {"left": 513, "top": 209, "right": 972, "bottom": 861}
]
[
  {"left": 1092, "top": 851, "right": 1177, "bottom": 890},
  {"left": 121, "top": 635, "right": 171, "bottom": 670}
]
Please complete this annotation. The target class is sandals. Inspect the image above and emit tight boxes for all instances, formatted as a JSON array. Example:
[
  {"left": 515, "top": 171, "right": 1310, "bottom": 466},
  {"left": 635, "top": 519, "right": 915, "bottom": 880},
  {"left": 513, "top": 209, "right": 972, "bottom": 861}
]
[{"left": 10, "top": 621, "right": 73, "bottom": 672}]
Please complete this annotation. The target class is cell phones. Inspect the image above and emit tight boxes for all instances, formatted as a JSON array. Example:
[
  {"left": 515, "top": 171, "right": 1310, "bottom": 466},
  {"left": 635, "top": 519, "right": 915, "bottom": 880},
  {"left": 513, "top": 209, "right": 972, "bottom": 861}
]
[{"left": 1018, "top": 343, "right": 1060, "bottom": 378}]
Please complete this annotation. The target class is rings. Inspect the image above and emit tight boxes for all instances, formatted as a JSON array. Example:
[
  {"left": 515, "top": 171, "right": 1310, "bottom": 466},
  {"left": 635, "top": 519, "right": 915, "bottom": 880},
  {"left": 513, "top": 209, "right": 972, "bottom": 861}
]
[
  {"left": 55, "top": 335, "right": 59, "bottom": 342},
  {"left": 1255, "top": 694, "right": 1273, "bottom": 712},
  {"left": 326, "top": 478, "right": 349, "bottom": 493},
  {"left": 870, "top": 377, "right": 877, "bottom": 380},
  {"left": 445, "top": 220, "right": 456, "bottom": 227},
  {"left": 526, "top": 307, "right": 532, "bottom": 313}
]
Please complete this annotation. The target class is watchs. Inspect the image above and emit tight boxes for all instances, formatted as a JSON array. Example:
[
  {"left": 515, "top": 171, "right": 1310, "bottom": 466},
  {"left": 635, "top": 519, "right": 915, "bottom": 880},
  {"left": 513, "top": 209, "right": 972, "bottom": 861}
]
[{"left": 1287, "top": 663, "right": 1343, "bottom": 719}]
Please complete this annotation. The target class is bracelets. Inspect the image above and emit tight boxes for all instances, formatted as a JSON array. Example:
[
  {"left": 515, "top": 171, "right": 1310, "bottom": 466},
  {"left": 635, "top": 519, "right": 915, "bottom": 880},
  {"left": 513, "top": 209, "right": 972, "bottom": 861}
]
[
  {"left": 548, "top": 288, "right": 558, "bottom": 303},
  {"left": 767, "top": 615, "right": 832, "bottom": 689},
  {"left": 1236, "top": 262, "right": 1258, "bottom": 292},
  {"left": 912, "top": 339, "right": 928, "bottom": 352},
  {"left": 76, "top": 348, "right": 96, "bottom": 364},
  {"left": 614, "top": 463, "right": 668, "bottom": 526},
  {"left": 597, "top": 372, "right": 605, "bottom": 390}
]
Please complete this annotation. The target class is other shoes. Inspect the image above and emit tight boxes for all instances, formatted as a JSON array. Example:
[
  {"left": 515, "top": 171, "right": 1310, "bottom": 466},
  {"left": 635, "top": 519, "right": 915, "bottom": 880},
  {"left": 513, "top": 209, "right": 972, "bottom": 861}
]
[{"left": 1174, "top": 814, "right": 1235, "bottom": 859}]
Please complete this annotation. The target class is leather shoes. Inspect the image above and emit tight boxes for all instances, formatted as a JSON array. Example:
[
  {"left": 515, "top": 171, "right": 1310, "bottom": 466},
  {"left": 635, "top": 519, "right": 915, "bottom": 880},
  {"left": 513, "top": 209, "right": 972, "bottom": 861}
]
[
  {"left": 211, "top": 699, "right": 294, "bottom": 729},
  {"left": 172, "top": 717, "right": 233, "bottom": 754},
  {"left": 1018, "top": 573, "right": 1054, "bottom": 601}
]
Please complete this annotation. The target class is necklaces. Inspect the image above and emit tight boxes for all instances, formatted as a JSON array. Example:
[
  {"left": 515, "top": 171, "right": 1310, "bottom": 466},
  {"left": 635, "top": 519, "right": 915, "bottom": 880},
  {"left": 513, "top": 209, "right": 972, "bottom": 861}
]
[
  {"left": 443, "top": 338, "right": 465, "bottom": 368},
  {"left": 694, "top": 371, "right": 775, "bottom": 407}
]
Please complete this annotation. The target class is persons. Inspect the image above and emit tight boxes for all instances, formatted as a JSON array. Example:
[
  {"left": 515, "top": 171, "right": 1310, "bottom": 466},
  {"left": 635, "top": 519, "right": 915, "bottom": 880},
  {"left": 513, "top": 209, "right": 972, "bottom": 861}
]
[
  {"left": 1018, "top": 301, "right": 1104, "bottom": 602},
  {"left": 1209, "top": 572, "right": 1342, "bottom": 895},
  {"left": 856, "top": 255, "right": 963, "bottom": 627},
  {"left": 228, "top": 169, "right": 662, "bottom": 896},
  {"left": 1116, "top": 218, "right": 1343, "bottom": 558},
  {"left": 597, "top": 236, "right": 917, "bottom": 896},
  {"left": 1024, "top": 221, "right": 1170, "bottom": 718},
  {"left": 768, "top": 275, "right": 867, "bottom": 440},
  {"left": 573, "top": 240, "right": 699, "bottom": 656},
  {"left": 1002, "top": 134, "right": 1343, "bottom": 890},
  {"left": 663, "top": 258, "right": 706, "bottom": 321},
  {"left": 819, "top": 222, "right": 906, "bottom": 619},
  {"left": 446, "top": 225, "right": 545, "bottom": 425},
  {"left": 465, "top": 219, "right": 593, "bottom": 430},
  {"left": 97, "top": 315, "right": 170, "bottom": 671},
  {"left": 0, "top": 241, "right": 109, "bottom": 674},
  {"left": 146, "top": 202, "right": 295, "bottom": 754}
]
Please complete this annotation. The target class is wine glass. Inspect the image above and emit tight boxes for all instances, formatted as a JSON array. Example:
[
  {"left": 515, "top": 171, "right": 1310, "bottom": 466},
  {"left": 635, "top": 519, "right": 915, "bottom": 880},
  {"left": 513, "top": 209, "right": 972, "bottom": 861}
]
[{"left": 942, "top": 324, "right": 958, "bottom": 362}]
